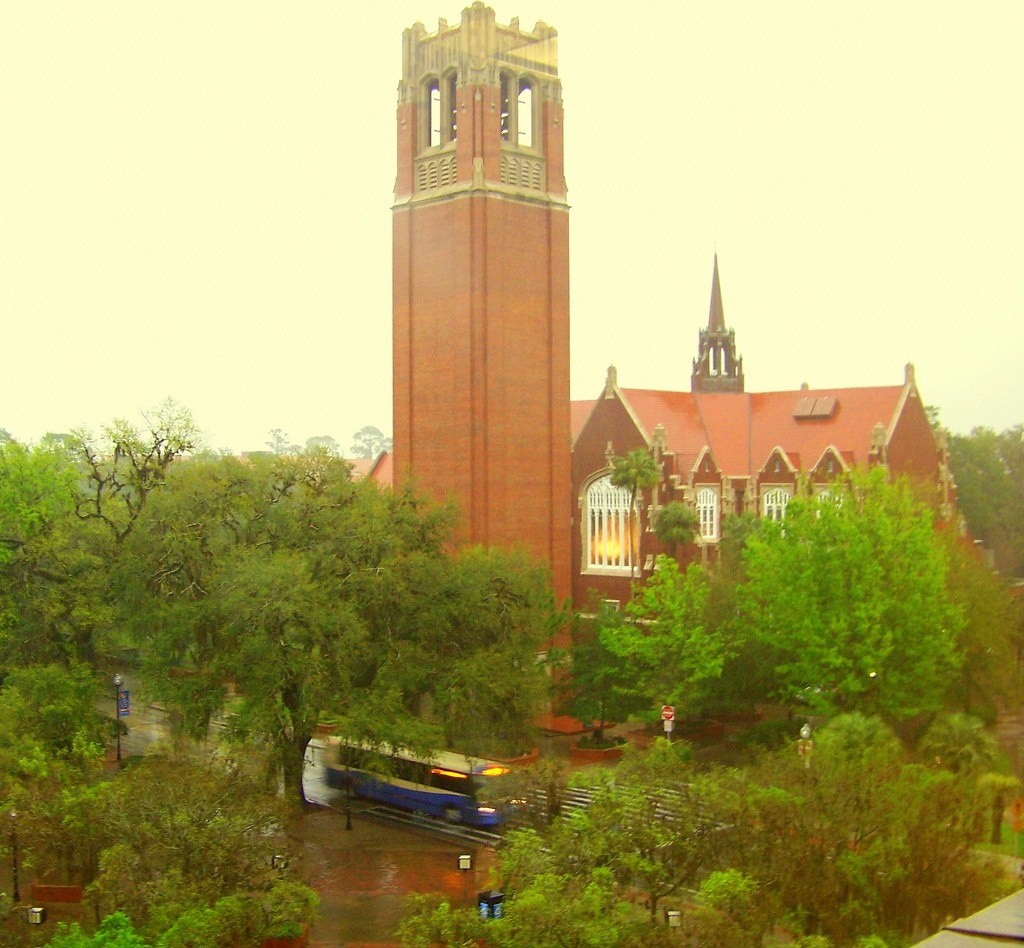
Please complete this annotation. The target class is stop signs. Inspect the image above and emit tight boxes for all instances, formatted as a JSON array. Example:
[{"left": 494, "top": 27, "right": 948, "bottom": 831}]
[
  {"left": 661, "top": 706, "right": 675, "bottom": 720},
  {"left": 799, "top": 741, "right": 813, "bottom": 755}
]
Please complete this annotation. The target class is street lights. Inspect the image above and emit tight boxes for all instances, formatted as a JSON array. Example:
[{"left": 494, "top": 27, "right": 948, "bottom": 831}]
[
  {"left": 27, "top": 906, "right": 48, "bottom": 931},
  {"left": 799, "top": 725, "right": 814, "bottom": 779},
  {"left": 10, "top": 809, "right": 20, "bottom": 901},
  {"left": 112, "top": 674, "right": 124, "bottom": 761},
  {"left": 339, "top": 728, "right": 354, "bottom": 830},
  {"left": 458, "top": 855, "right": 472, "bottom": 904}
]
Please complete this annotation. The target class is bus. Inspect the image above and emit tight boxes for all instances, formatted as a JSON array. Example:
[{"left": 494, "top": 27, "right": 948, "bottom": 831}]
[{"left": 324, "top": 735, "right": 519, "bottom": 825}]
